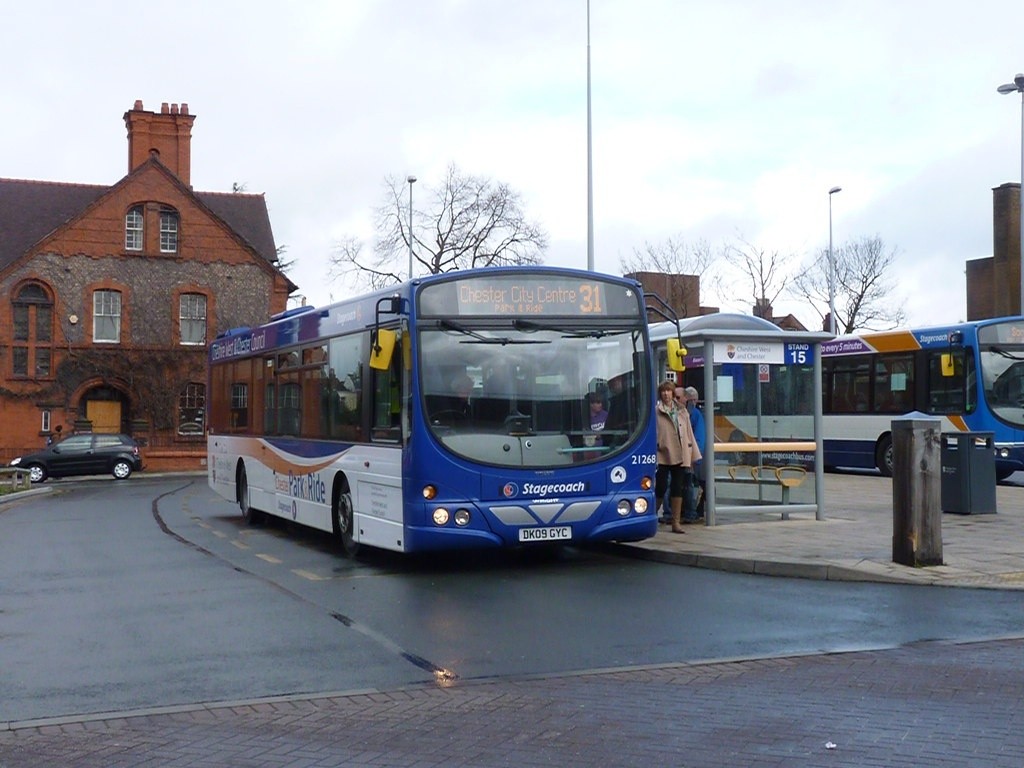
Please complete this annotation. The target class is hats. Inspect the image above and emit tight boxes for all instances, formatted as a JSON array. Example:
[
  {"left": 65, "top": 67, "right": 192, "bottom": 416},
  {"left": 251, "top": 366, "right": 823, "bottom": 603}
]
[{"left": 585, "top": 393, "right": 604, "bottom": 403}]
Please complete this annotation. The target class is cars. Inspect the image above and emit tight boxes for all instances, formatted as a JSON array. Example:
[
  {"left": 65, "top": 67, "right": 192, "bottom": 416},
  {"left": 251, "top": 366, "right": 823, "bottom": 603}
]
[{"left": 178, "top": 422, "right": 204, "bottom": 436}]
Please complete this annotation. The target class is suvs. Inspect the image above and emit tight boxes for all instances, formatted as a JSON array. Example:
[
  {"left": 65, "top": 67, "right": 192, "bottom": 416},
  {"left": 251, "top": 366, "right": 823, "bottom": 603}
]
[{"left": 6, "top": 432, "right": 142, "bottom": 484}]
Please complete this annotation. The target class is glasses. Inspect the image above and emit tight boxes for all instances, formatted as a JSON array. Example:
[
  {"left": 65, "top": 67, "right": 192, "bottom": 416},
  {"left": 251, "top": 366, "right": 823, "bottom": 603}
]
[{"left": 676, "top": 396, "right": 680, "bottom": 399}]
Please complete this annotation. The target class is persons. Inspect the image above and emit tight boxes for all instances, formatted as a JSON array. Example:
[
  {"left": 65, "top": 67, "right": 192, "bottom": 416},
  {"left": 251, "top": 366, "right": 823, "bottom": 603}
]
[
  {"left": 436, "top": 376, "right": 488, "bottom": 421},
  {"left": 655, "top": 380, "right": 706, "bottom": 534},
  {"left": 590, "top": 393, "right": 608, "bottom": 455},
  {"left": 597, "top": 370, "right": 637, "bottom": 451}
]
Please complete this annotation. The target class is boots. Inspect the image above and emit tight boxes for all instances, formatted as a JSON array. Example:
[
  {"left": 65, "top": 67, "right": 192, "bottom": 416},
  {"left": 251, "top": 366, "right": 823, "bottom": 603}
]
[
  {"left": 670, "top": 498, "right": 687, "bottom": 535},
  {"left": 655, "top": 498, "right": 663, "bottom": 516}
]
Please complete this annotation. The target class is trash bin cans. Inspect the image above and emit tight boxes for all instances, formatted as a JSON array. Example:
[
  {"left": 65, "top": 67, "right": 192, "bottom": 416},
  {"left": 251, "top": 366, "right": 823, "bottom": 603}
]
[{"left": 940, "top": 431, "right": 999, "bottom": 515}]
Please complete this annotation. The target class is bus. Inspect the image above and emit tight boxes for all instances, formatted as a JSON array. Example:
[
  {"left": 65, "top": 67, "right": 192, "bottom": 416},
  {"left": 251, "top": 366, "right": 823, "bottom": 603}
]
[
  {"left": 207, "top": 266, "right": 688, "bottom": 564},
  {"left": 675, "top": 316, "right": 1024, "bottom": 483}
]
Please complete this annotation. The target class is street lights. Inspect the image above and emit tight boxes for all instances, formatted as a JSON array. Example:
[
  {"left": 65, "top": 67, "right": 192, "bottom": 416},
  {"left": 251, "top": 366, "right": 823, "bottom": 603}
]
[
  {"left": 407, "top": 173, "right": 418, "bottom": 279},
  {"left": 997, "top": 73, "right": 1024, "bottom": 318},
  {"left": 828, "top": 185, "right": 843, "bottom": 336}
]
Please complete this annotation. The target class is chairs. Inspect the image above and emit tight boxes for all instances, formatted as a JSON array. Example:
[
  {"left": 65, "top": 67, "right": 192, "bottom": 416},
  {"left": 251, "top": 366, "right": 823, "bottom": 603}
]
[{"left": 232, "top": 384, "right": 563, "bottom": 432}]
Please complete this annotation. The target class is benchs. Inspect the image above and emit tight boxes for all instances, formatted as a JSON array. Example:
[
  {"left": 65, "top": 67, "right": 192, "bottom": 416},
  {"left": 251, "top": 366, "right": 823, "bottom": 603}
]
[{"left": 714, "top": 467, "right": 807, "bottom": 505}]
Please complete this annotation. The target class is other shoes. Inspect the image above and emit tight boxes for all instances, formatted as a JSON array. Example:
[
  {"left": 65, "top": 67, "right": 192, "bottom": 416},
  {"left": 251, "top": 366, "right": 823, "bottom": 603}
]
[{"left": 659, "top": 516, "right": 674, "bottom": 523}]
